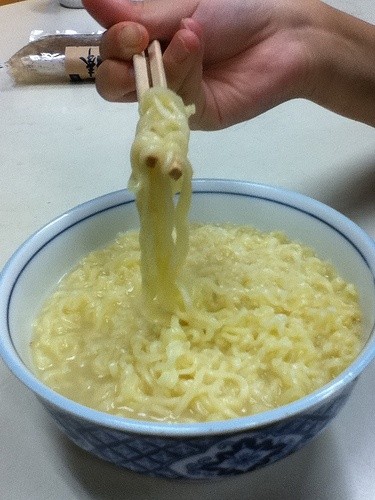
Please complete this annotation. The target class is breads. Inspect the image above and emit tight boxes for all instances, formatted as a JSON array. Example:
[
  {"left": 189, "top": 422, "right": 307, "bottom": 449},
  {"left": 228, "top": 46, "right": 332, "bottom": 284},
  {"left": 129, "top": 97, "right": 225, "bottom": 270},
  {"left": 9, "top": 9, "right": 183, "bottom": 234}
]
[{"left": 3, "top": 33, "right": 108, "bottom": 84}]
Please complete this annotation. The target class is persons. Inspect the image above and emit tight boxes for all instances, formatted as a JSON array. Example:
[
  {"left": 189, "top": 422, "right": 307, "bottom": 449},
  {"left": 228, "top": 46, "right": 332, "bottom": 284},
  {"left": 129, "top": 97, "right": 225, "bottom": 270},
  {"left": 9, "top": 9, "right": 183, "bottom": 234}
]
[{"left": 81, "top": 1, "right": 374, "bottom": 132}]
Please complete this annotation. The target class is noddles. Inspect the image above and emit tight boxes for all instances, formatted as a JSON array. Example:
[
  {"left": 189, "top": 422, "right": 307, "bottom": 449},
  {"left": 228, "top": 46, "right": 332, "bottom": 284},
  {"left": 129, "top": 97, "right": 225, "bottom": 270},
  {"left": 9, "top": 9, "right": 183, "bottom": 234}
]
[{"left": 31, "top": 87, "right": 361, "bottom": 425}]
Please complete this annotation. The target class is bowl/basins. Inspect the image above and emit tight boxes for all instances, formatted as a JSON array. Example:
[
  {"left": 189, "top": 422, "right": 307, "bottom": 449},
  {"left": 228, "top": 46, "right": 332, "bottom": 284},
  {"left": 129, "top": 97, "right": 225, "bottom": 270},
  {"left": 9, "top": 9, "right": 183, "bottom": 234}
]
[{"left": 2, "top": 179, "right": 370, "bottom": 481}]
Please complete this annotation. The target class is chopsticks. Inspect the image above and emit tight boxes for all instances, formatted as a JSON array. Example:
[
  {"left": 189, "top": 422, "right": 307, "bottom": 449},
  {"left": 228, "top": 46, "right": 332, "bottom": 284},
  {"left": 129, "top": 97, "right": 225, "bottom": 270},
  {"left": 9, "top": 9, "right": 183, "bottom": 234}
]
[{"left": 134, "top": 36, "right": 182, "bottom": 183}]
